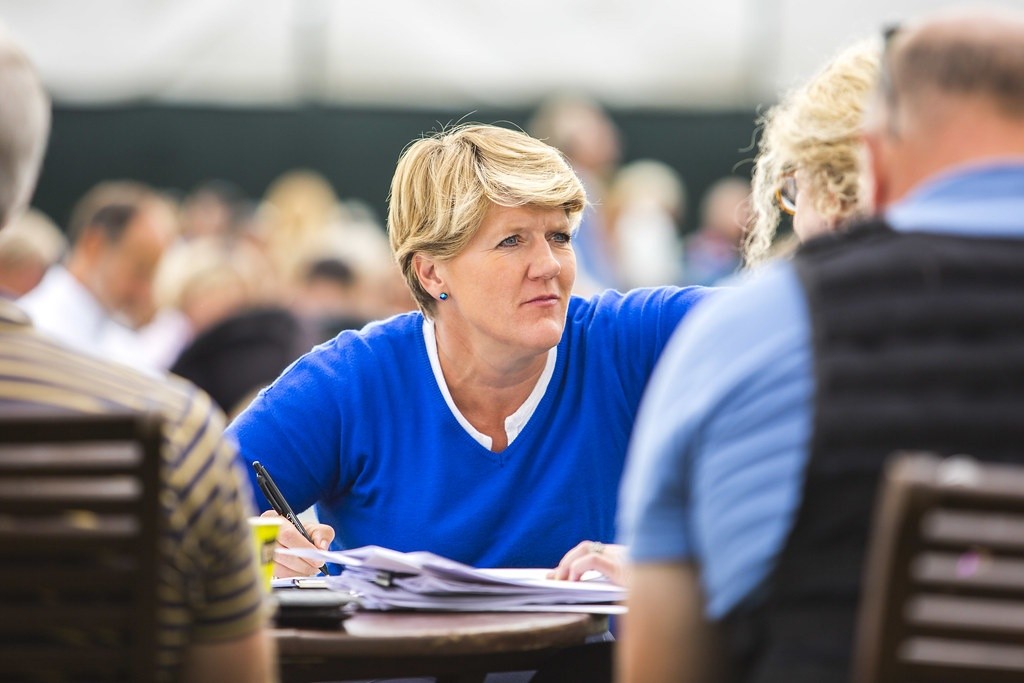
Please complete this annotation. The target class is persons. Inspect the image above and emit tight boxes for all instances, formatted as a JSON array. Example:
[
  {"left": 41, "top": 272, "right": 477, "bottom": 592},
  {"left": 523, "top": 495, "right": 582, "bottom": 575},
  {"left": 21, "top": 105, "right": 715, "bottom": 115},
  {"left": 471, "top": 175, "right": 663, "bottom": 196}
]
[
  {"left": 221, "top": 121, "right": 740, "bottom": 580},
  {"left": 0, "top": 53, "right": 419, "bottom": 683},
  {"left": 530, "top": 13, "right": 1024, "bottom": 683}
]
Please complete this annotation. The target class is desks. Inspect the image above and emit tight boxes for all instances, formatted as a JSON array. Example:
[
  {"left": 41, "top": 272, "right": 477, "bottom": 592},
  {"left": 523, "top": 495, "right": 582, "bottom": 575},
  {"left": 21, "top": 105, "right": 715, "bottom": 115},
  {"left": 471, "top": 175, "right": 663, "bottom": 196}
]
[{"left": 270, "top": 609, "right": 610, "bottom": 683}]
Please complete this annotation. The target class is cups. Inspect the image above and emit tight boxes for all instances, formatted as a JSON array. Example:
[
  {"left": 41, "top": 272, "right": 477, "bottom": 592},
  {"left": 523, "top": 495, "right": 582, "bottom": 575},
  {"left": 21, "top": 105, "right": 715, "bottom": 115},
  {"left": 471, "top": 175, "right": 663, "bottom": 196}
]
[{"left": 247, "top": 517, "right": 284, "bottom": 595}]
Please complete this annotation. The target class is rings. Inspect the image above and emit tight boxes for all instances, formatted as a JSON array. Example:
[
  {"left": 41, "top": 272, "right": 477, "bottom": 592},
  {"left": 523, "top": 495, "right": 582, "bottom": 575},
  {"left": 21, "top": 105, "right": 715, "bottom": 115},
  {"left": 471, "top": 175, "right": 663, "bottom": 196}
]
[{"left": 592, "top": 541, "right": 605, "bottom": 554}]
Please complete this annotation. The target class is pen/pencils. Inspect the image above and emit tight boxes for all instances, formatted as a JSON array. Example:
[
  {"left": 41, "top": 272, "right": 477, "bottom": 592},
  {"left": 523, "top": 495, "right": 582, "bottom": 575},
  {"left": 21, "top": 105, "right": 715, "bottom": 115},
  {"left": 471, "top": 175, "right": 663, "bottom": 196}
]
[{"left": 251, "top": 458, "right": 332, "bottom": 576}]
